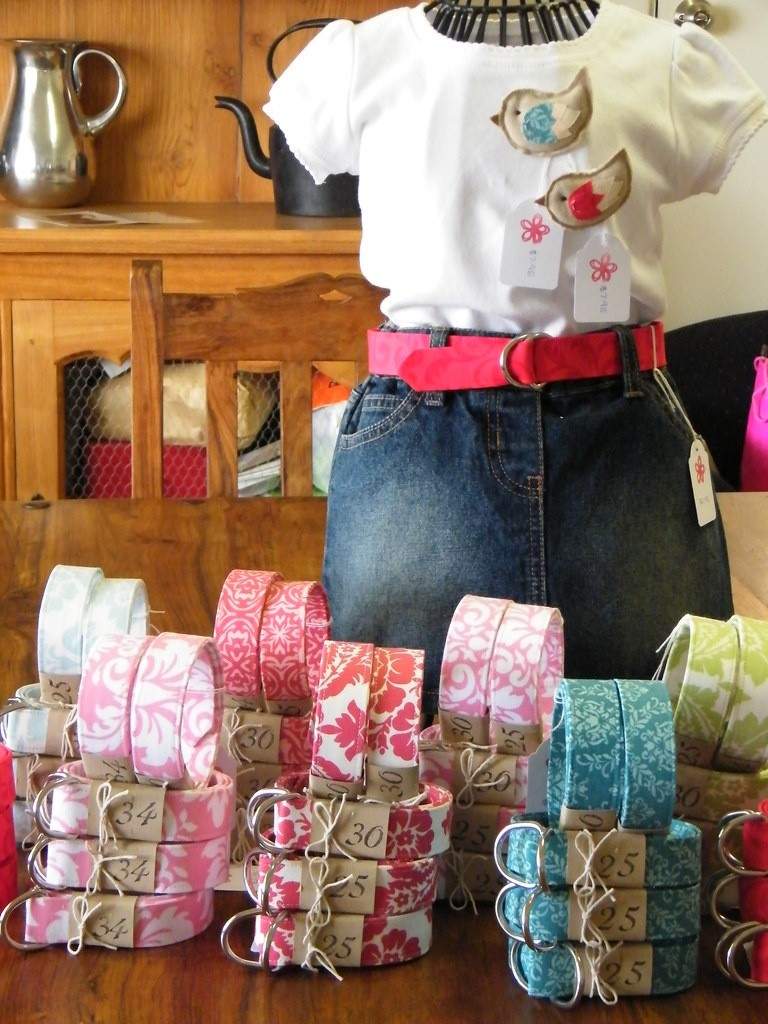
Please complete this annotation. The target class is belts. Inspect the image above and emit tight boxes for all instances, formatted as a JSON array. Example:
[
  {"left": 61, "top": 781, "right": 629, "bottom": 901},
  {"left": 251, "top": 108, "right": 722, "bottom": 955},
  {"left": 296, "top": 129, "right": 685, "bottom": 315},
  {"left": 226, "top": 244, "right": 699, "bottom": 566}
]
[
  {"left": 0, "top": 561, "right": 768, "bottom": 999},
  {"left": 368, "top": 321, "right": 667, "bottom": 392}
]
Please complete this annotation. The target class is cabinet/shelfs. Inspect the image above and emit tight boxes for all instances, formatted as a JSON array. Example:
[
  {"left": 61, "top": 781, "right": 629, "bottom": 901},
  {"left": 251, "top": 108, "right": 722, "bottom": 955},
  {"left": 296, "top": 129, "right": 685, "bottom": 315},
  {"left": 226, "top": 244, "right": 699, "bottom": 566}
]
[{"left": 0, "top": 204, "right": 362, "bottom": 501}]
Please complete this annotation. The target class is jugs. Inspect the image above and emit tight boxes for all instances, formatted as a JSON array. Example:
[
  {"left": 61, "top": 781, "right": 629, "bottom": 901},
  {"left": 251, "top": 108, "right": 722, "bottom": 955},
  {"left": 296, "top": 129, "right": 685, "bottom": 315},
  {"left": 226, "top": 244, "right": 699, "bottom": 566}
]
[{"left": 0, "top": 32, "right": 127, "bottom": 207}]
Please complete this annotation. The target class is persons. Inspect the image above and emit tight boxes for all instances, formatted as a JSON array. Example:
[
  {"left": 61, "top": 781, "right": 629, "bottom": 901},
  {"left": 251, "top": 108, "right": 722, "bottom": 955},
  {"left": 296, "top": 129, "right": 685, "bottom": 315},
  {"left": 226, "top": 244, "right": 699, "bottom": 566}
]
[{"left": 263, "top": 0, "right": 766, "bottom": 676}]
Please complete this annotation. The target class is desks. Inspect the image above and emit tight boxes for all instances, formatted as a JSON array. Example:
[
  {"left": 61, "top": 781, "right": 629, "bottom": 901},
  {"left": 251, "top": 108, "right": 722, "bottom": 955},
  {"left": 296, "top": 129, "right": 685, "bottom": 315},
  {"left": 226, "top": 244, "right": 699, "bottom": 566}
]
[{"left": 0, "top": 492, "right": 768, "bottom": 1024}]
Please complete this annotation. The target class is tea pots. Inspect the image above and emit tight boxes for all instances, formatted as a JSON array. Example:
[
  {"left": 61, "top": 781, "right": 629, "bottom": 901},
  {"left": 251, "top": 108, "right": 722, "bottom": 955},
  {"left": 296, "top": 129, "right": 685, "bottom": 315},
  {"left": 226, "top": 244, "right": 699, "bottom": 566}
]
[{"left": 216, "top": 19, "right": 357, "bottom": 218}]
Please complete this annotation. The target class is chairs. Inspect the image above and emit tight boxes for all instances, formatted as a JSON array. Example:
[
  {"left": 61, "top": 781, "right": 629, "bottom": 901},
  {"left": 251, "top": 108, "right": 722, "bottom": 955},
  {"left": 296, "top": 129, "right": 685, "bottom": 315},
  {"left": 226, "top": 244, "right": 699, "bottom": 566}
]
[{"left": 129, "top": 258, "right": 385, "bottom": 499}]
